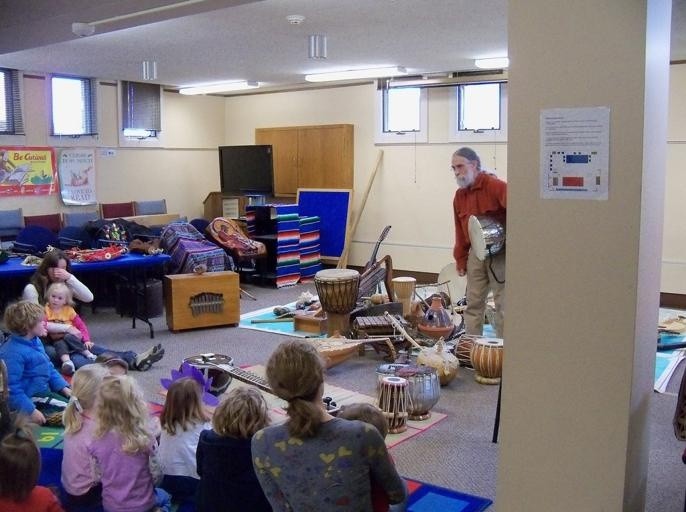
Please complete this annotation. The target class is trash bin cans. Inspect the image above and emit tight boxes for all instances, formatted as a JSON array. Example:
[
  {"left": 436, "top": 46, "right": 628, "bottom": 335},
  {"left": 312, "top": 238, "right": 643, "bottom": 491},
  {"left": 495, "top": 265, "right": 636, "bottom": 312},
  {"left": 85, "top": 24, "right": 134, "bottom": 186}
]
[{"left": 138, "top": 278, "right": 163, "bottom": 318}]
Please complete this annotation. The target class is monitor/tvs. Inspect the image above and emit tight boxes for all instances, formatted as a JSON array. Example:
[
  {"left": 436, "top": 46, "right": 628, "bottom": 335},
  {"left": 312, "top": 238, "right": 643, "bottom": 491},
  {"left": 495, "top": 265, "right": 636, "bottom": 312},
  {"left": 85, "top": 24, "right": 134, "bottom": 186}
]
[{"left": 218, "top": 144, "right": 275, "bottom": 197}]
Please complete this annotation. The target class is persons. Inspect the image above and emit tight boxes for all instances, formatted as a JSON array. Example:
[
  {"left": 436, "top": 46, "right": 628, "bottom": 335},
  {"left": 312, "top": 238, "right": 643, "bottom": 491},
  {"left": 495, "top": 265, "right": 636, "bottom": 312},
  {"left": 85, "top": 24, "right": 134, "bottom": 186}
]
[
  {"left": 195, "top": 387, "right": 272, "bottom": 511},
  {"left": 1, "top": 425, "right": 63, "bottom": 512},
  {"left": 450, "top": 146, "right": 508, "bottom": 340},
  {"left": 19, "top": 252, "right": 166, "bottom": 371},
  {"left": 334, "top": 401, "right": 389, "bottom": 443},
  {"left": 84, "top": 374, "right": 172, "bottom": 512},
  {"left": 158, "top": 377, "right": 214, "bottom": 510},
  {"left": 61, "top": 361, "right": 112, "bottom": 511},
  {"left": 251, "top": 338, "right": 405, "bottom": 511},
  {"left": 1, "top": 301, "right": 88, "bottom": 426},
  {"left": 42, "top": 281, "right": 97, "bottom": 371}
]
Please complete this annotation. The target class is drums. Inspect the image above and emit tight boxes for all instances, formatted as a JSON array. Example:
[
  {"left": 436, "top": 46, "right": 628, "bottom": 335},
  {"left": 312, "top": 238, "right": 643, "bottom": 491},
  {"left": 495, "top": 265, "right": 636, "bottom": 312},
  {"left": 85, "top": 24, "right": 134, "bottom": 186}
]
[
  {"left": 455, "top": 334, "right": 479, "bottom": 367},
  {"left": 398, "top": 365, "right": 438, "bottom": 420},
  {"left": 314, "top": 267, "right": 360, "bottom": 338},
  {"left": 371, "top": 377, "right": 416, "bottom": 433},
  {"left": 471, "top": 337, "right": 504, "bottom": 384},
  {"left": 390, "top": 277, "right": 417, "bottom": 316},
  {"left": 468, "top": 213, "right": 506, "bottom": 263}
]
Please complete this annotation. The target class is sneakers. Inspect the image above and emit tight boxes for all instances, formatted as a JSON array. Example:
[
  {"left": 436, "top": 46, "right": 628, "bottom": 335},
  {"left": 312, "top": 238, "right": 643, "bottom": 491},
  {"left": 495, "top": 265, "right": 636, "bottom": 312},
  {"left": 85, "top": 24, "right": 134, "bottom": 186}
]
[
  {"left": 62, "top": 360, "right": 75, "bottom": 375},
  {"left": 135, "top": 344, "right": 164, "bottom": 371}
]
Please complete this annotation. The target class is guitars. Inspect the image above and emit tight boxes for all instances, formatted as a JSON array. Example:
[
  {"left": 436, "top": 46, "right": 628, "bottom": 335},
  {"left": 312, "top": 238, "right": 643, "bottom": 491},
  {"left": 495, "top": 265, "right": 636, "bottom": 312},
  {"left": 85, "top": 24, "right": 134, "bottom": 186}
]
[{"left": 361, "top": 225, "right": 391, "bottom": 297}]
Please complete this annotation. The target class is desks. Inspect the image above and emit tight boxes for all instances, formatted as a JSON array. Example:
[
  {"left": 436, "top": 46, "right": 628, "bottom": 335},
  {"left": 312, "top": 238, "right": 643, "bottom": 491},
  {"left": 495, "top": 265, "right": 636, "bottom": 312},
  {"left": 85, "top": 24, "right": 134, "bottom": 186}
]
[{"left": 0, "top": 248, "right": 172, "bottom": 338}]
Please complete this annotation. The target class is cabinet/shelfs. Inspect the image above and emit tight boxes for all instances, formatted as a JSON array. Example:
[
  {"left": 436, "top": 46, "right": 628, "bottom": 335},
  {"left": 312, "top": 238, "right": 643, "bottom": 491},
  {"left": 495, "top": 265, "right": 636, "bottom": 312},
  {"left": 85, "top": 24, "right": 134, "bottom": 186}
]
[{"left": 255, "top": 124, "right": 354, "bottom": 204}]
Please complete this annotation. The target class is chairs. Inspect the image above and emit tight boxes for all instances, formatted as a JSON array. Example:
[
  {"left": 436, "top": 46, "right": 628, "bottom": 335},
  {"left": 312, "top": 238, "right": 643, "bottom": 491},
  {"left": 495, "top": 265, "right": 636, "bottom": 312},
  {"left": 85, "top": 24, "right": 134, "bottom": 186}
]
[{"left": 0, "top": 198, "right": 168, "bottom": 316}]
